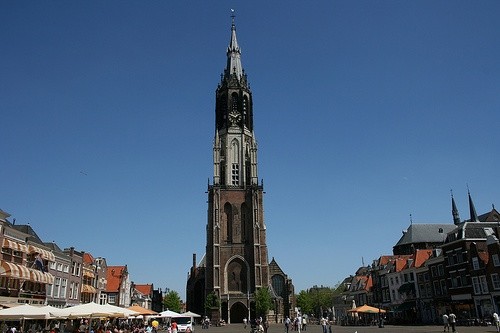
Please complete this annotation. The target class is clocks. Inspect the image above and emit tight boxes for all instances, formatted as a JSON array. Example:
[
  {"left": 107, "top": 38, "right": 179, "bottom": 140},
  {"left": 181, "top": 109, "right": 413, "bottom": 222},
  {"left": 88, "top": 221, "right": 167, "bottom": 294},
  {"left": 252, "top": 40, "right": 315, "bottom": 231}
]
[{"left": 227, "top": 109, "right": 242, "bottom": 124}]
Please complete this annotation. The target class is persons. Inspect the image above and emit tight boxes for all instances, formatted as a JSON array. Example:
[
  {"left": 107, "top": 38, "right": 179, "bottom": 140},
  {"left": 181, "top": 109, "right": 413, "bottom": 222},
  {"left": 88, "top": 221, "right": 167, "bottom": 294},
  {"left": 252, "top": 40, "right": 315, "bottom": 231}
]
[
  {"left": 152, "top": 319, "right": 159, "bottom": 333},
  {"left": 493, "top": 311, "right": 500, "bottom": 333},
  {"left": 284, "top": 314, "right": 306, "bottom": 333},
  {"left": 321, "top": 317, "right": 330, "bottom": 333},
  {"left": 171, "top": 320, "right": 177, "bottom": 333},
  {"left": 258, "top": 325, "right": 264, "bottom": 333},
  {"left": 168, "top": 326, "right": 171, "bottom": 333},
  {"left": 243, "top": 317, "right": 247, "bottom": 328},
  {"left": 249, "top": 318, "right": 259, "bottom": 332},
  {"left": 0, "top": 319, "right": 151, "bottom": 333},
  {"left": 443, "top": 312, "right": 450, "bottom": 332},
  {"left": 449, "top": 312, "right": 456, "bottom": 332},
  {"left": 201, "top": 317, "right": 209, "bottom": 329}
]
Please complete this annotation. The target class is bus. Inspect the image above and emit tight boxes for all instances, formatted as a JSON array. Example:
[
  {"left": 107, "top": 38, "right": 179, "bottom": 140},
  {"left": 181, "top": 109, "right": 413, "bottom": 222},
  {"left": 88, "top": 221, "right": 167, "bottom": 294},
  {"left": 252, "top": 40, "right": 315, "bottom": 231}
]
[{"left": 169, "top": 316, "right": 196, "bottom": 333}]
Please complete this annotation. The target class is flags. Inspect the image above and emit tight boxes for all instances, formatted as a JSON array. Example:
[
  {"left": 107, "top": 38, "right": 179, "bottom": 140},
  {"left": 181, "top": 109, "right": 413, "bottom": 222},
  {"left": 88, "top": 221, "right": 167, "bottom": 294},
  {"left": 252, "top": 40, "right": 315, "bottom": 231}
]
[{"left": 35, "top": 256, "right": 44, "bottom": 274}]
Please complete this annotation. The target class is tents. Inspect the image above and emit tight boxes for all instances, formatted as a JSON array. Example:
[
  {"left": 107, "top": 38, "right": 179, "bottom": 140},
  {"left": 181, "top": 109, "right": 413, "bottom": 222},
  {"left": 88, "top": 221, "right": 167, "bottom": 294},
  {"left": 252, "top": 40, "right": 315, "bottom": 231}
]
[{"left": 346, "top": 304, "right": 387, "bottom": 326}]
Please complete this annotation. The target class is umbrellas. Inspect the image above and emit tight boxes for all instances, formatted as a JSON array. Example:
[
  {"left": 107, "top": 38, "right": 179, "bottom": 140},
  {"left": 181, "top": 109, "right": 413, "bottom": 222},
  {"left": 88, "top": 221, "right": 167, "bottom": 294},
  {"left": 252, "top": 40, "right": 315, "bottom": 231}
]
[
  {"left": 158, "top": 309, "right": 201, "bottom": 320},
  {"left": 0, "top": 301, "right": 162, "bottom": 318}
]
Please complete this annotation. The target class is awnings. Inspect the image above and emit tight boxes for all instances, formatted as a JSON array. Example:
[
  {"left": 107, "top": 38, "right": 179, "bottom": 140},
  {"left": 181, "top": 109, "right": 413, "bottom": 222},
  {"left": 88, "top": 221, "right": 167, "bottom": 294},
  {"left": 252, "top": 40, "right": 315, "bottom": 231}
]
[
  {"left": 3, "top": 238, "right": 28, "bottom": 254},
  {"left": 82, "top": 266, "right": 95, "bottom": 278},
  {"left": 81, "top": 284, "right": 97, "bottom": 294},
  {"left": 0, "top": 260, "right": 30, "bottom": 280},
  {"left": 28, "top": 268, "right": 54, "bottom": 284},
  {"left": 28, "top": 245, "right": 55, "bottom": 263}
]
